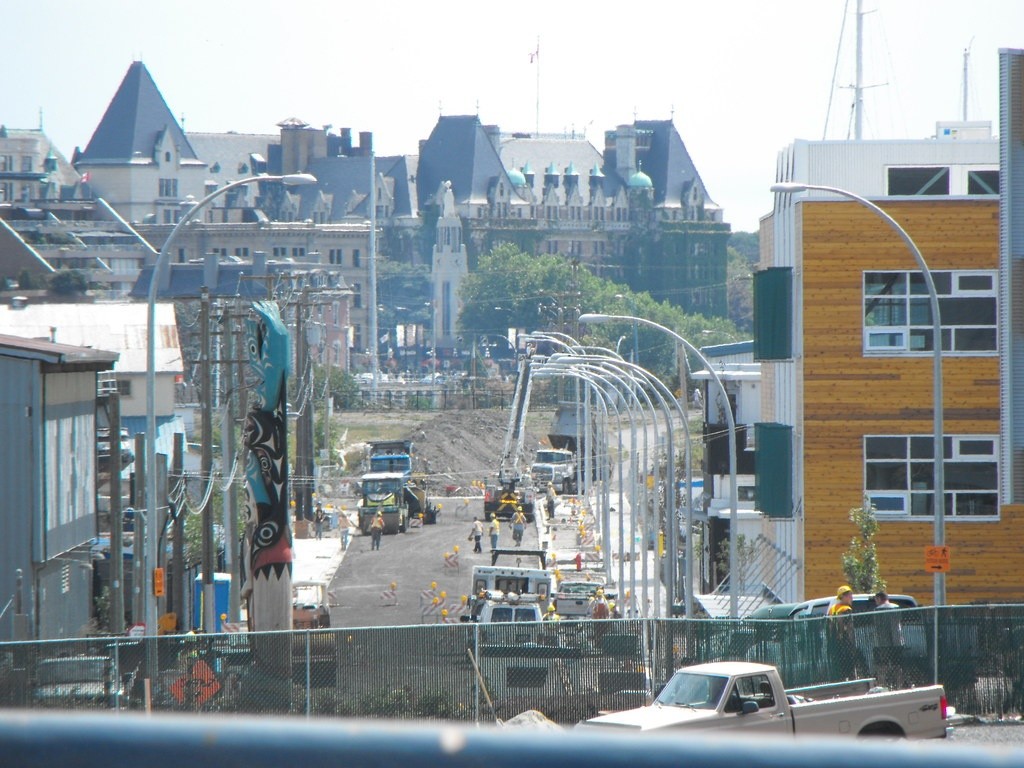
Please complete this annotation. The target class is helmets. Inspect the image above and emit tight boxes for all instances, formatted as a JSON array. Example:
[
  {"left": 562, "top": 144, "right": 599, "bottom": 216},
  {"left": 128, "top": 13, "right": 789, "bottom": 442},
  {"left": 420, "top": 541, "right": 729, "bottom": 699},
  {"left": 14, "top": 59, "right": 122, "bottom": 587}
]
[
  {"left": 317, "top": 501, "right": 321, "bottom": 506},
  {"left": 376, "top": 510, "right": 383, "bottom": 517},
  {"left": 597, "top": 589, "right": 603, "bottom": 594},
  {"left": 547, "top": 482, "right": 552, "bottom": 485},
  {"left": 517, "top": 506, "right": 522, "bottom": 510},
  {"left": 490, "top": 512, "right": 495, "bottom": 517},
  {"left": 547, "top": 604, "right": 555, "bottom": 612},
  {"left": 836, "top": 586, "right": 852, "bottom": 600},
  {"left": 608, "top": 603, "right": 615, "bottom": 610}
]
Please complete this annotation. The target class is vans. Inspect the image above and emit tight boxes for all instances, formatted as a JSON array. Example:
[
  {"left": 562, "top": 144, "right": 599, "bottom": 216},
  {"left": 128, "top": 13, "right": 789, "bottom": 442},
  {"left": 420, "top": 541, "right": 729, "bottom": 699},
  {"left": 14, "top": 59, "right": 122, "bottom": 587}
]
[
  {"left": 730, "top": 594, "right": 928, "bottom": 663},
  {"left": 460, "top": 591, "right": 545, "bottom": 645},
  {"left": 473, "top": 565, "right": 550, "bottom": 612}
]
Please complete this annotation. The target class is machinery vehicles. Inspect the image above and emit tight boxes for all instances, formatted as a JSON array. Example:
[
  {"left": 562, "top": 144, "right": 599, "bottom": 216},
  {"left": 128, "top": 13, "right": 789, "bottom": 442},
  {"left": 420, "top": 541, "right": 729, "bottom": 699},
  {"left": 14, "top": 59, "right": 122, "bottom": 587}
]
[
  {"left": 484, "top": 350, "right": 537, "bottom": 522},
  {"left": 357, "top": 440, "right": 426, "bottom": 535},
  {"left": 291, "top": 581, "right": 333, "bottom": 629}
]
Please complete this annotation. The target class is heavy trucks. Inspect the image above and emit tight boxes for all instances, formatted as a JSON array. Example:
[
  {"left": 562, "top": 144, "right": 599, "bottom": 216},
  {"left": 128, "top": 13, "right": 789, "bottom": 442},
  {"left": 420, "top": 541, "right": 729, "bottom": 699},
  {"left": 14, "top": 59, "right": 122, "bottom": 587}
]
[{"left": 530, "top": 401, "right": 615, "bottom": 491}]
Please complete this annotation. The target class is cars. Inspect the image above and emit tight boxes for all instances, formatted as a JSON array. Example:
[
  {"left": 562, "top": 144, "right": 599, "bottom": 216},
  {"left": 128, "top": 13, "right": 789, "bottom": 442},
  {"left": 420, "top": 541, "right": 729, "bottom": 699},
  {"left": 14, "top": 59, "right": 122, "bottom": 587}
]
[{"left": 38, "top": 657, "right": 127, "bottom": 712}]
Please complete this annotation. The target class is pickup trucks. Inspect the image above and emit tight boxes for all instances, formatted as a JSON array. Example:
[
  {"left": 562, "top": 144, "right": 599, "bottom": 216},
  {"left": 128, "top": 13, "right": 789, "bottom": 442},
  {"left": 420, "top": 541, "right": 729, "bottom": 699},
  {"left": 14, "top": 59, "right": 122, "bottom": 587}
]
[
  {"left": 552, "top": 582, "right": 614, "bottom": 616},
  {"left": 574, "top": 663, "right": 949, "bottom": 741}
]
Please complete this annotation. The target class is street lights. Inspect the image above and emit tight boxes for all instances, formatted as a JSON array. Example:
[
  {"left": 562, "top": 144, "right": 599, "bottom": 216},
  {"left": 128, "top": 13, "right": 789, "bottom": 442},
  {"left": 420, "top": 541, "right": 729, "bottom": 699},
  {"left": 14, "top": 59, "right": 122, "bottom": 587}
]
[
  {"left": 145, "top": 175, "right": 319, "bottom": 676},
  {"left": 770, "top": 182, "right": 950, "bottom": 607},
  {"left": 518, "top": 314, "right": 739, "bottom": 659}
]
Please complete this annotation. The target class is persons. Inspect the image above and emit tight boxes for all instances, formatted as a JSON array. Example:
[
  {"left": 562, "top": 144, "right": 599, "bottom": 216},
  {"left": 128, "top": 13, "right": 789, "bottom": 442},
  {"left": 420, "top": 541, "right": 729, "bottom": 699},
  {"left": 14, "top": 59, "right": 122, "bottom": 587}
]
[
  {"left": 542, "top": 604, "right": 561, "bottom": 646},
  {"left": 338, "top": 511, "right": 349, "bottom": 551},
  {"left": 468, "top": 516, "right": 484, "bottom": 554},
  {"left": 508, "top": 506, "right": 527, "bottom": 547},
  {"left": 588, "top": 587, "right": 618, "bottom": 650},
  {"left": 825, "top": 585, "right": 869, "bottom": 680},
  {"left": 872, "top": 592, "right": 906, "bottom": 690},
  {"left": 311, "top": 501, "right": 325, "bottom": 541},
  {"left": 369, "top": 511, "right": 384, "bottom": 551},
  {"left": 545, "top": 481, "right": 557, "bottom": 522},
  {"left": 488, "top": 513, "right": 499, "bottom": 549},
  {"left": 691, "top": 386, "right": 703, "bottom": 409}
]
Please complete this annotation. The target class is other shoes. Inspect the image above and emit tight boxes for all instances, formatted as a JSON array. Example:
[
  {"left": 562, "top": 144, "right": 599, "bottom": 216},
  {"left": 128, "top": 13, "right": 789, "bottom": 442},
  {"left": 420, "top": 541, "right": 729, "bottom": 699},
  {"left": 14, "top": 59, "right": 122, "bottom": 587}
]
[
  {"left": 316, "top": 536, "right": 321, "bottom": 540},
  {"left": 546, "top": 515, "right": 554, "bottom": 521},
  {"left": 473, "top": 549, "right": 481, "bottom": 554},
  {"left": 515, "top": 543, "right": 520, "bottom": 546},
  {"left": 340, "top": 548, "right": 345, "bottom": 551},
  {"left": 371, "top": 547, "right": 379, "bottom": 550}
]
